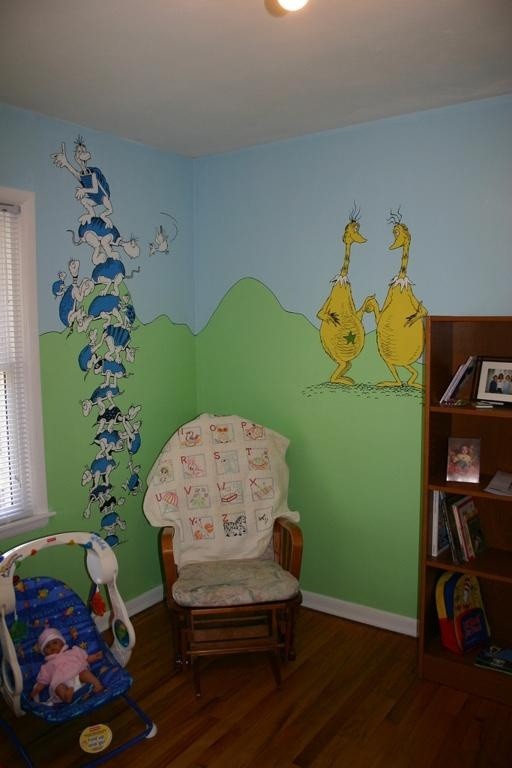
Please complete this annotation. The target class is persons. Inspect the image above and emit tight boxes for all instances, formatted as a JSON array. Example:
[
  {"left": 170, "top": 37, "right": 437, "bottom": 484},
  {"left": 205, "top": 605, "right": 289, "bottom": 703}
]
[{"left": 29, "top": 625, "right": 106, "bottom": 703}]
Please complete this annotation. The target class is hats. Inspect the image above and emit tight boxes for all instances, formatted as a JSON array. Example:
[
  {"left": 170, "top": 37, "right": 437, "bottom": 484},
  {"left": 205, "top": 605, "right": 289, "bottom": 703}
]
[{"left": 37, "top": 627, "right": 68, "bottom": 655}]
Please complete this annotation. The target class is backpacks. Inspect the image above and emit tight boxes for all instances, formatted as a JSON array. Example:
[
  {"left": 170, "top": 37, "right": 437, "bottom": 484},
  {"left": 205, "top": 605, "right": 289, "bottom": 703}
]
[{"left": 433, "top": 570, "right": 494, "bottom": 657}]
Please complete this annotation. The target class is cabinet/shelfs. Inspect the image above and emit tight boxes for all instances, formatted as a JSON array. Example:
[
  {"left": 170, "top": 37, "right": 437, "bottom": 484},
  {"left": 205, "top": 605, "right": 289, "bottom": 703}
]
[{"left": 416, "top": 313, "right": 512, "bottom": 703}]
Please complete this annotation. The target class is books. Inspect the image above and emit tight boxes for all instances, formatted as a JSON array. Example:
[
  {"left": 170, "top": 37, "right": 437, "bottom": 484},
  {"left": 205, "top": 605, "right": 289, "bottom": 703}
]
[
  {"left": 474, "top": 644, "right": 511, "bottom": 675},
  {"left": 426, "top": 354, "right": 512, "bottom": 565}
]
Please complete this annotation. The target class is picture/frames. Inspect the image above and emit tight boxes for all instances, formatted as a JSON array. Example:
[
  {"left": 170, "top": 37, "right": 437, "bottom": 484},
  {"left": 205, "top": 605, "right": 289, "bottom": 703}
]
[
  {"left": 469, "top": 355, "right": 512, "bottom": 409},
  {"left": 437, "top": 430, "right": 486, "bottom": 489}
]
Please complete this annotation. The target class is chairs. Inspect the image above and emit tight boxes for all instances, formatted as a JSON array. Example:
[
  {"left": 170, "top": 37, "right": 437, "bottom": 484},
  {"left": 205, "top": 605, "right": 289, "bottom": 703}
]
[{"left": 159, "top": 414, "right": 305, "bottom": 699}]
